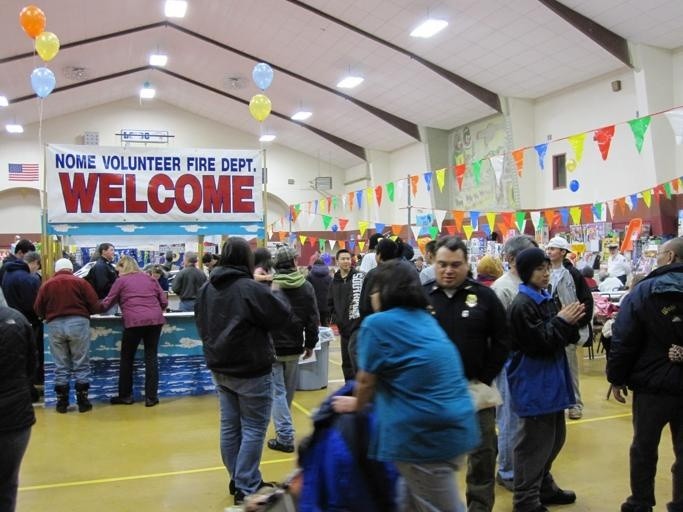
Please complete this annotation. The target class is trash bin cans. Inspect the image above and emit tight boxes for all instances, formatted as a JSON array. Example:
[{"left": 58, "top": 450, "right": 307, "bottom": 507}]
[{"left": 297, "top": 326, "right": 334, "bottom": 390}]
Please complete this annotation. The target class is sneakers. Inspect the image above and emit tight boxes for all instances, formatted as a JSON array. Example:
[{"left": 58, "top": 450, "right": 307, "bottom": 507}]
[
  {"left": 235, "top": 480, "right": 273, "bottom": 505},
  {"left": 541, "top": 482, "right": 575, "bottom": 504},
  {"left": 621, "top": 501, "right": 652, "bottom": 512},
  {"left": 496, "top": 471, "right": 515, "bottom": 491},
  {"left": 268, "top": 439, "right": 294, "bottom": 452},
  {"left": 569, "top": 405, "right": 582, "bottom": 419}
]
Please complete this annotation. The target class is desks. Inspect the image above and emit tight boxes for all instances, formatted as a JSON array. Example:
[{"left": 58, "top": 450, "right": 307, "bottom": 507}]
[{"left": 42, "top": 311, "right": 204, "bottom": 358}]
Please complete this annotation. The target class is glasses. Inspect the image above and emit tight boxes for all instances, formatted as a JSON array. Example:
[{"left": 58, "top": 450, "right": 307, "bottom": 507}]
[{"left": 533, "top": 265, "right": 553, "bottom": 273}]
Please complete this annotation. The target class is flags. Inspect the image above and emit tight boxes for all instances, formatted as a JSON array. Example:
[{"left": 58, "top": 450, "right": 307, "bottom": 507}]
[{"left": 8, "top": 162, "right": 40, "bottom": 183}]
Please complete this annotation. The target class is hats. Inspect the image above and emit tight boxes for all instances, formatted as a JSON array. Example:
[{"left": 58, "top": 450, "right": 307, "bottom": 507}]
[
  {"left": 276, "top": 246, "right": 298, "bottom": 262},
  {"left": 55, "top": 258, "right": 73, "bottom": 273},
  {"left": 607, "top": 243, "right": 618, "bottom": 248},
  {"left": 547, "top": 237, "right": 571, "bottom": 252}
]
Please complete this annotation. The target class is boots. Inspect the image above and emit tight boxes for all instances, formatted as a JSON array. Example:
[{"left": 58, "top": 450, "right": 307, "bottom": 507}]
[
  {"left": 75, "top": 383, "right": 92, "bottom": 412},
  {"left": 55, "top": 383, "right": 70, "bottom": 412}
]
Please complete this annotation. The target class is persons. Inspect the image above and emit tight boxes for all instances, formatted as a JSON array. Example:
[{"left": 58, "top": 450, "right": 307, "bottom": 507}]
[
  {"left": 1, "top": 288, "right": 44, "bottom": 511},
  {"left": 192, "top": 236, "right": 277, "bottom": 502},
  {"left": 0, "top": 239, "right": 218, "bottom": 414},
  {"left": 242, "top": 233, "right": 595, "bottom": 511},
  {"left": 583, "top": 236, "right": 649, "bottom": 303},
  {"left": 605, "top": 236, "right": 683, "bottom": 512}
]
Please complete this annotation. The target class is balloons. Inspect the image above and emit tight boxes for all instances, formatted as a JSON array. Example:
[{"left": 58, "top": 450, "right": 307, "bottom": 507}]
[
  {"left": 31, "top": 67, "right": 56, "bottom": 99},
  {"left": 251, "top": 61, "right": 273, "bottom": 91},
  {"left": 248, "top": 93, "right": 273, "bottom": 122},
  {"left": 34, "top": 31, "right": 59, "bottom": 63},
  {"left": 18, "top": 6, "right": 45, "bottom": 39}
]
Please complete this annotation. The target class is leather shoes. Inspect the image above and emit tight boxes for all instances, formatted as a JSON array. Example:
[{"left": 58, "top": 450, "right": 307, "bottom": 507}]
[
  {"left": 111, "top": 396, "right": 133, "bottom": 404},
  {"left": 146, "top": 398, "right": 159, "bottom": 405}
]
